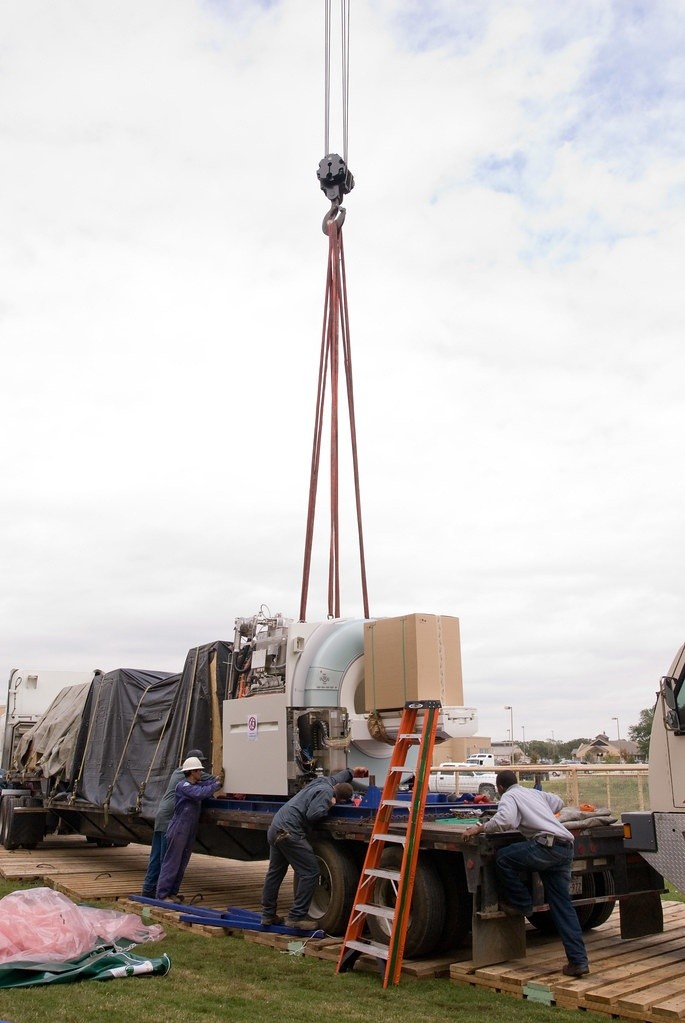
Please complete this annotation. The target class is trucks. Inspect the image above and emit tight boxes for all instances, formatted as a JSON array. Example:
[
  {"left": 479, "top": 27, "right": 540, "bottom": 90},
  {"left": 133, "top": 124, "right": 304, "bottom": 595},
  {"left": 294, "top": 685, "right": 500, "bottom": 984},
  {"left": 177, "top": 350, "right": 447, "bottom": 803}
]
[{"left": 466, "top": 754, "right": 494, "bottom": 774}]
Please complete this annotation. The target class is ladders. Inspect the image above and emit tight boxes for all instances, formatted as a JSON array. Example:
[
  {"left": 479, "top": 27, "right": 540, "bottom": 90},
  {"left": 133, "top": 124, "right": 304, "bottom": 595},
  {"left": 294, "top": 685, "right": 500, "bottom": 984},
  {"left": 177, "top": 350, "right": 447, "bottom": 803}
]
[{"left": 332, "top": 698, "right": 444, "bottom": 993}]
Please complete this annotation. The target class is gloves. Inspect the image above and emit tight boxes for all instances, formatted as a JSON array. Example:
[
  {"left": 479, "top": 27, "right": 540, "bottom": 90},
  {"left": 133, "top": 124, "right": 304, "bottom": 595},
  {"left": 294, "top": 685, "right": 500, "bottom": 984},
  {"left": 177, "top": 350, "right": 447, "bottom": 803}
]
[{"left": 218, "top": 768, "right": 225, "bottom": 785}]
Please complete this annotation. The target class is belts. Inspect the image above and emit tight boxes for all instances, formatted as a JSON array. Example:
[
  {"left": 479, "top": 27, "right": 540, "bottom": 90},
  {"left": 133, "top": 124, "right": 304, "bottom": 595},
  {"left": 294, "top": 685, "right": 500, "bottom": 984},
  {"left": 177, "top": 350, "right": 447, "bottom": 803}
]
[{"left": 556, "top": 840, "right": 573, "bottom": 849}]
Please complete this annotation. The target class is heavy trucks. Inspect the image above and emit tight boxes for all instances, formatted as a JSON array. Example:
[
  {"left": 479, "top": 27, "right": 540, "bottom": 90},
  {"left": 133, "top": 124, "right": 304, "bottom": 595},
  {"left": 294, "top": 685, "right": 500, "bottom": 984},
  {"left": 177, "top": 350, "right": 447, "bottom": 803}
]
[{"left": 0, "top": 602, "right": 660, "bottom": 965}]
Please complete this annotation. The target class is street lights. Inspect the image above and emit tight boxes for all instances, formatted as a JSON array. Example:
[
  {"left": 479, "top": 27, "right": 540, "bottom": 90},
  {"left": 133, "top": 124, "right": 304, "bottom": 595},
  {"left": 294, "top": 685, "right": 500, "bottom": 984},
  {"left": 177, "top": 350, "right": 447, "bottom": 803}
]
[
  {"left": 500, "top": 702, "right": 528, "bottom": 764},
  {"left": 611, "top": 716, "right": 623, "bottom": 764}
]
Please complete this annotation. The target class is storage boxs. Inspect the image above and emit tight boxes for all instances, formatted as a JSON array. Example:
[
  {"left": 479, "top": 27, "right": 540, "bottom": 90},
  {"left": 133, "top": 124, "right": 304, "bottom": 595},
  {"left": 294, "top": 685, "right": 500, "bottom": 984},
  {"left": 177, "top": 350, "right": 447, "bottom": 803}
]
[{"left": 362, "top": 612, "right": 463, "bottom": 710}]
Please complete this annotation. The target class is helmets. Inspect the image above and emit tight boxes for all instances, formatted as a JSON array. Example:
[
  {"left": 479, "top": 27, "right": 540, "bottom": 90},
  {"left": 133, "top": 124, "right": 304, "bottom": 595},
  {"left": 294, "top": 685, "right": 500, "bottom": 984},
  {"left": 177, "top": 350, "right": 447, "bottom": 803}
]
[{"left": 181, "top": 757, "right": 205, "bottom": 771}]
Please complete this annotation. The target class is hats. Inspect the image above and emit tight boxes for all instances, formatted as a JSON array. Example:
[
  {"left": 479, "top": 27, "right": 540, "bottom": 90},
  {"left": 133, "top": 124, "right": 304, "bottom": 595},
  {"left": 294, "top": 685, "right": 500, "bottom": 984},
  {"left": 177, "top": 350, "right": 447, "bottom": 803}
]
[{"left": 187, "top": 750, "right": 209, "bottom": 761}]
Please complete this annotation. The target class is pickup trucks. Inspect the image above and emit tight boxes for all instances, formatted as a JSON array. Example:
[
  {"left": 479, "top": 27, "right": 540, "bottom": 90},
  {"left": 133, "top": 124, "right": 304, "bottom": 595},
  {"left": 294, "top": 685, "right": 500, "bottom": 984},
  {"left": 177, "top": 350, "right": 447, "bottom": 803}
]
[{"left": 428, "top": 761, "right": 501, "bottom": 801}]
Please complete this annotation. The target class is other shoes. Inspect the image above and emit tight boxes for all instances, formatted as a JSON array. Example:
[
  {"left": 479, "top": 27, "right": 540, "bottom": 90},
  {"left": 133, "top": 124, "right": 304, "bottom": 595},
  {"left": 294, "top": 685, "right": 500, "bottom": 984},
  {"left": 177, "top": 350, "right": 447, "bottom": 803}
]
[
  {"left": 563, "top": 961, "right": 589, "bottom": 975},
  {"left": 162, "top": 894, "right": 180, "bottom": 903},
  {"left": 285, "top": 919, "right": 319, "bottom": 930},
  {"left": 261, "top": 916, "right": 285, "bottom": 925},
  {"left": 499, "top": 901, "right": 532, "bottom": 917}
]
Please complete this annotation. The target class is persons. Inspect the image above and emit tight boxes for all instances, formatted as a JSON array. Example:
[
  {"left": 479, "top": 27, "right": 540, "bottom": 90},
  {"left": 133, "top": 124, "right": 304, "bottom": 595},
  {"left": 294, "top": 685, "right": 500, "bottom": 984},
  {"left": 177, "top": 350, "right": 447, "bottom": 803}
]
[
  {"left": 156, "top": 758, "right": 225, "bottom": 904},
  {"left": 260, "top": 768, "right": 353, "bottom": 930},
  {"left": 142, "top": 749, "right": 218, "bottom": 898},
  {"left": 461, "top": 771, "right": 590, "bottom": 977},
  {"left": 534, "top": 778, "right": 543, "bottom": 791}
]
